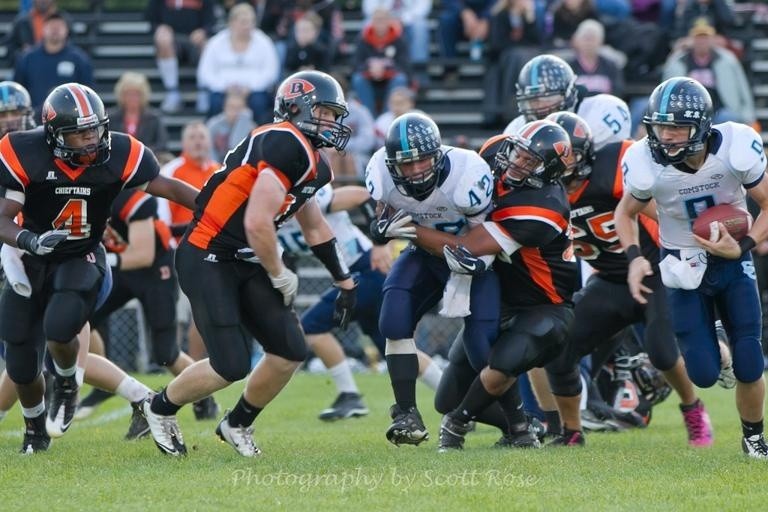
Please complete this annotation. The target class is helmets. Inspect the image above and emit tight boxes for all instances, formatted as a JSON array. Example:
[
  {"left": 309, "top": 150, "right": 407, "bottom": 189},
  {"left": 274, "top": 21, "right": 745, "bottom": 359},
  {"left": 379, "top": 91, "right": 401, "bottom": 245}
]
[
  {"left": 41, "top": 82, "right": 112, "bottom": 168},
  {"left": 642, "top": 76, "right": 714, "bottom": 165},
  {"left": 273, "top": 69, "right": 353, "bottom": 157},
  {"left": 633, "top": 351, "right": 672, "bottom": 405},
  {"left": 0, "top": 80, "right": 37, "bottom": 138},
  {"left": 492, "top": 54, "right": 594, "bottom": 189},
  {"left": 384, "top": 113, "right": 453, "bottom": 197}
]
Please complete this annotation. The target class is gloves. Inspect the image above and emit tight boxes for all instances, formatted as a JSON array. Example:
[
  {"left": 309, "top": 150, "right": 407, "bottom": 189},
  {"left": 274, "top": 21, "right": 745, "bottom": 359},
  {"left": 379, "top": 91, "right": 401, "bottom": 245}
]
[
  {"left": 16, "top": 229, "right": 71, "bottom": 256},
  {"left": 443, "top": 244, "right": 485, "bottom": 275},
  {"left": 269, "top": 265, "right": 299, "bottom": 306},
  {"left": 333, "top": 280, "right": 360, "bottom": 330},
  {"left": 370, "top": 202, "right": 417, "bottom": 244}
]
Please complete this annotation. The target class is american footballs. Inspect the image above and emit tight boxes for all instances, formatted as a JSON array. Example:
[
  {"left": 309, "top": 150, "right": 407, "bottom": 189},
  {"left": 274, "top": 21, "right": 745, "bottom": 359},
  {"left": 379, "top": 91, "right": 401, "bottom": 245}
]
[{"left": 691, "top": 204, "right": 752, "bottom": 241}]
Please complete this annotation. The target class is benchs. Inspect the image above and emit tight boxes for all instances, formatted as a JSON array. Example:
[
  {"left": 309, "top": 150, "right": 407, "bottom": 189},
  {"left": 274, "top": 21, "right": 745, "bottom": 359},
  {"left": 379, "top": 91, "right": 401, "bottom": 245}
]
[{"left": 1, "top": 0, "right": 767, "bottom": 226}]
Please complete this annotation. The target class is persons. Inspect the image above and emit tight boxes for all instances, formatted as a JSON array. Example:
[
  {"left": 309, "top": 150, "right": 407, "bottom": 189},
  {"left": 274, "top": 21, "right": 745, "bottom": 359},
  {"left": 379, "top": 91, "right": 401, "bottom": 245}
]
[{"left": 0, "top": 1, "right": 767, "bottom": 463}]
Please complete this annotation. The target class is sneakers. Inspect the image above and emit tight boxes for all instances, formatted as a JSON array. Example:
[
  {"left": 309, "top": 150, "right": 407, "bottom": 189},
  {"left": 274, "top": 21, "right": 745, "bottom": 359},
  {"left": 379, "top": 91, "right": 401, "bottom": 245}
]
[
  {"left": 385, "top": 403, "right": 430, "bottom": 448},
  {"left": 580, "top": 409, "right": 620, "bottom": 432},
  {"left": 75, "top": 388, "right": 116, "bottom": 420},
  {"left": 682, "top": 400, "right": 712, "bottom": 446},
  {"left": 125, "top": 390, "right": 187, "bottom": 456},
  {"left": 437, "top": 412, "right": 476, "bottom": 453},
  {"left": 214, "top": 408, "right": 261, "bottom": 457},
  {"left": 44, "top": 371, "right": 78, "bottom": 438},
  {"left": 742, "top": 431, "right": 768, "bottom": 458},
  {"left": 319, "top": 391, "right": 368, "bottom": 421},
  {"left": 192, "top": 396, "right": 216, "bottom": 420},
  {"left": 489, "top": 415, "right": 585, "bottom": 447},
  {"left": 20, "top": 410, "right": 51, "bottom": 455},
  {"left": 715, "top": 319, "right": 736, "bottom": 388}
]
